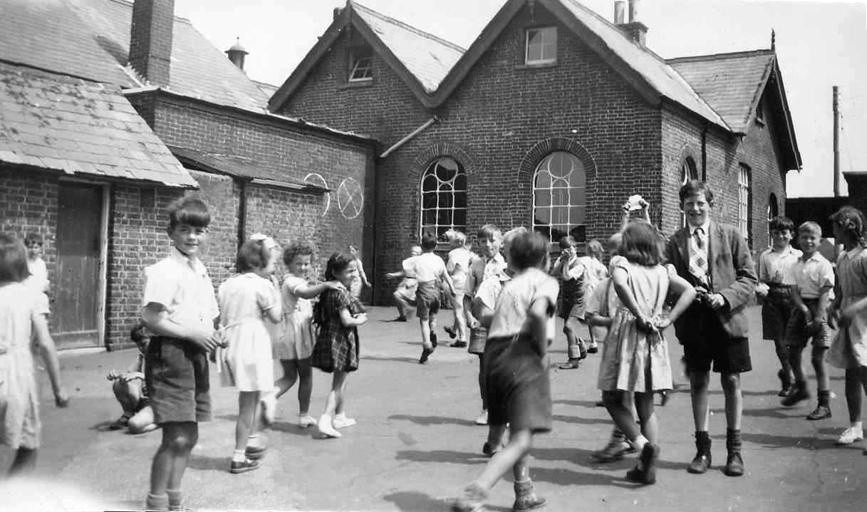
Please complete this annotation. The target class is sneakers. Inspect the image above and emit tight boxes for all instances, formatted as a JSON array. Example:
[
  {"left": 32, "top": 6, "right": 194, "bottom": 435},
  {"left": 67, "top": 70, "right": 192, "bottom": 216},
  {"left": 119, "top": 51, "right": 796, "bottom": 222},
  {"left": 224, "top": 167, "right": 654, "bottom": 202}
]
[
  {"left": 333, "top": 418, "right": 356, "bottom": 428},
  {"left": 623, "top": 441, "right": 633, "bottom": 453},
  {"left": 587, "top": 348, "right": 599, "bottom": 352},
  {"left": 590, "top": 443, "right": 622, "bottom": 463},
  {"left": 726, "top": 452, "right": 743, "bottom": 475},
  {"left": 626, "top": 467, "right": 642, "bottom": 479},
  {"left": 782, "top": 387, "right": 809, "bottom": 406},
  {"left": 806, "top": 404, "right": 832, "bottom": 420},
  {"left": 298, "top": 416, "right": 317, "bottom": 428},
  {"left": 318, "top": 421, "right": 341, "bottom": 438},
  {"left": 836, "top": 423, "right": 864, "bottom": 444},
  {"left": 430, "top": 331, "right": 437, "bottom": 347},
  {"left": 262, "top": 394, "right": 278, "bottom": 425},
  {"left": 688, "top": 452, "right": 712, "bottom": 473},
  {"left": 451, "top": 495, "right": 489, "bottom": 512},
  {"left": 420, "top": 347, "right": 433, "bottom": 362},
  {"left": 513, "top": 486, "right": 547, "bottom": 512},
  {"left": 660, "top": 389, "right": 670, "bottom": 405},
  {"left": 641, "top": 444, "right": 659, "bottom": 485},
  {"left": 231, "top": 459, "right": 259, "bottom": 472},
  {"left": 475, "top": 409, "right": 489, "bottom": 424},
  {"left": 559, "top": 357, "right": 578, "bottom": 369}
]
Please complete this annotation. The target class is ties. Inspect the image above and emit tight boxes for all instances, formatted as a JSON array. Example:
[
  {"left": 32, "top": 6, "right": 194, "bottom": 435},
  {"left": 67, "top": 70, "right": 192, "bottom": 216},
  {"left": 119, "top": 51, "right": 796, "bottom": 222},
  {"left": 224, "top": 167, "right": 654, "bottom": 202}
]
[{"left": 697, "top": 228, "right": 706, "bottom": 251}]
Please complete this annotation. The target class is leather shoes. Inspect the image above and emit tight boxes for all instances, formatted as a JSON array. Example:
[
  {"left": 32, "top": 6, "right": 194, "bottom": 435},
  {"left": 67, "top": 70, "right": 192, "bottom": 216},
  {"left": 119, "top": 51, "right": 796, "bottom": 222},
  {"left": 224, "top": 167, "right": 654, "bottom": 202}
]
[
  {"left": 578, "top": 353, "right": 586, "bottom": 359},
  {"left": 444, "top": 326, "right": 456, "bottom": 338},
  {"left": 779, "top": 381, "right": 796, "bottom": 397},
  {"left": 778, "top": 369, "right": 796, "bottom": 385},
  {"left": 450, "top": 340, "right": 464, "bottom": 347}
]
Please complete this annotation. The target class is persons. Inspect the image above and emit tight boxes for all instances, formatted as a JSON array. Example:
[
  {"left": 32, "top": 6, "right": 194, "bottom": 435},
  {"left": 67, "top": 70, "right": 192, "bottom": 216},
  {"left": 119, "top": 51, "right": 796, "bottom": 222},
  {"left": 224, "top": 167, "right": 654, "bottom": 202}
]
[
  {"left": 577, "top": 239, "right": 607, "bottom": 354},
  {"left": 401, "top": 234, "right": 456, "bottom": 365},
  {"left": 385, "top": 246, "right": 422, "bottom": 322},
  {"left": 24, "top": 232, "right": 53, "bottom": 322},
  {"left": 828, "top": 203, "right": 867, "bottom": 447},
  {"left": 346, "top": 242, "right": 372, "bottom": 299},
  {"left": 311, "top": 249, "right": 369, "bottom": 441},
  {"left": 462, "top": 222, "right": 506, "bottom": 425},
  {"left": 217, "top": 237, "right": 283, "bottom": 474},
  {"left": 597, "top": 220, "right": 698, "bottom": 488},
  {"left": 668, "top": 181, "right": 756, "bottom": 480},
  {"left": 620, "top": 198, "right": 653, "bottom": 230},
  {"left": 261, "top": 241, "right": 348, "bottom": 428},
  {"left": 589, "top": 233, "right": 635, "bottom": 459},
  {"left": 0, "top": 233, "right": 70, "bottom": 483},
  {"left": 443, "top": 232, "right": 480, "bottom": 348},
  {"left": 758, "top": 216, "right": 796, "bottom": 398},
  {"left": 780, "top": 220, "right": 833, "bottom": 420},
  {"left": 108, "top": 322, "right": 159, "bottom": 433},
  {"left": 450, "top": 234, "right": 561, "bottom": 512},
  {"left": 139, "top": 197, "right": 230, "bottom": 510},
  {"left": 551, "top": 235, "right": 587, "bottom": 369},
  {"left": 473, "top": 227, "right": 528, "bottom": 460}
]
